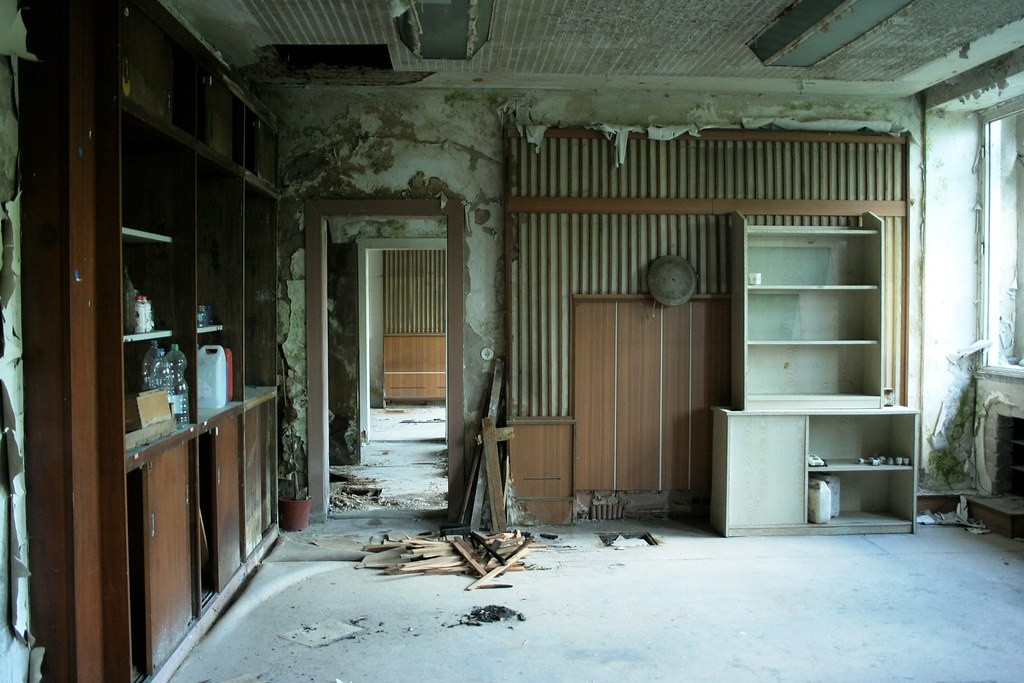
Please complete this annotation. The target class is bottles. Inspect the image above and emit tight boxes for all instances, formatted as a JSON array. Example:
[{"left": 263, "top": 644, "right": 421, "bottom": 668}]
[
  {"left": 165, "top": 343, "right": 189, "bottom": 429},
  {"left": 150, "top": 349, "right": 171, "bottom": 402},
  {"left": 123, "top": 266, "right": 153, "bottom": 335},
  {"left": 143, "top": 340, "right": 159, "bottom": 387}
]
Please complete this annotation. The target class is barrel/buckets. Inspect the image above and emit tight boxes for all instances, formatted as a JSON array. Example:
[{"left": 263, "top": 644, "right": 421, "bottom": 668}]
[
  {"left": 808, "top": 479, "right": 831, "bottom": 524},
  {"left": 816, "top": 472, "right": 841, "bottom": 520},
  {"left": 197, "top": 343, "right": 233, "bottom": 410},
  {"left": 281, "top": 497, "right": 310, "bottom": 531}
]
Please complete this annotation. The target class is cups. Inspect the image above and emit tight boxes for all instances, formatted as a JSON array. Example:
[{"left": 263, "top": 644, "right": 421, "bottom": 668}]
[{"left": 748, "top": 272, "right": 762, "bottom": 284}]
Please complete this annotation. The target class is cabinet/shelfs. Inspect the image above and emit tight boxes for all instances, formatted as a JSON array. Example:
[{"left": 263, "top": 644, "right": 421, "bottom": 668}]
[
  {"left": 711, "top": 211, "right": 920, "bottom": 538},
  {"left": 17, "top": 0, "right": 283, "bottom": 683},
  {"left": 507, "top": 415, "right": 573, "bottom": 524}
]
